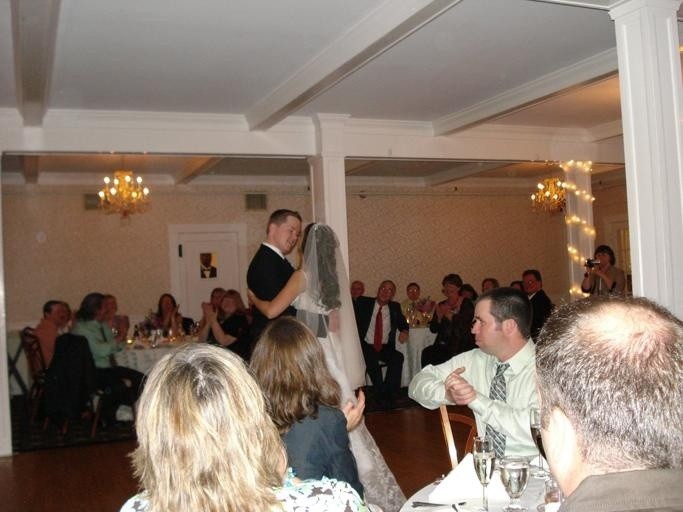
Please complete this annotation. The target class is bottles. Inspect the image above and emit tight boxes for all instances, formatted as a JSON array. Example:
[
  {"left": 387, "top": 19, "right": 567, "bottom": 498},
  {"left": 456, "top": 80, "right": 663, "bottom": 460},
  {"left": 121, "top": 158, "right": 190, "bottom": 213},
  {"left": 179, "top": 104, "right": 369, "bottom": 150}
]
[{"left": 132, "top": 324, "right": 138, "bottom": 340}]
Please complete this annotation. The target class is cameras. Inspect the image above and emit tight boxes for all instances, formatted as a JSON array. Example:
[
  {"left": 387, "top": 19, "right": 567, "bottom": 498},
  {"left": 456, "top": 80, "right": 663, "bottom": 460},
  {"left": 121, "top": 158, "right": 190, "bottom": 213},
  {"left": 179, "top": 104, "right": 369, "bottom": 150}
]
[{"left": 586, "top": 257, "right": 601, "bottom": 267}]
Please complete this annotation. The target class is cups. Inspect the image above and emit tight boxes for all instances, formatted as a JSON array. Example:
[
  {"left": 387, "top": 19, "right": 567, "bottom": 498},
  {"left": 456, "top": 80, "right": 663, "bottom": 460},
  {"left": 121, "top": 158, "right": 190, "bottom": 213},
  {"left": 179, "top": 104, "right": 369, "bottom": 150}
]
[
  {"left": 543, "top": 478, "right": 563, "bottom": 504},
  {"left": 184, "top": 336, "right": 192, "bottom": 342},
  {"left": 409, "top": 315, "right": 427, "bottom": 327},
  {"left": 528, "top": 407, "right": 556, "bottom": 461}
]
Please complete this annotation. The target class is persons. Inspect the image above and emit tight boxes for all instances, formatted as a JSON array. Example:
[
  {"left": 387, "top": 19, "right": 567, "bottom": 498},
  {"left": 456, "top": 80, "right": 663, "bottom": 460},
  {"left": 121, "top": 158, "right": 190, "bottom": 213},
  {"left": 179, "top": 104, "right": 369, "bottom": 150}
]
[
  {"left": 581, "top": 245, "right": 625, "bottom": 295},
  {"left": 533, "top": 292, "right": 683, "bottom": 512},
  {"left": 201, "top": 254, "right": 216, "bottom": 279},
  {"left": 120, "top": 341, "right": 371, "bottom": 512},
  {"left": 247, "top": 315, "right": 367, "bottom": 501},
  {"left": 350, "top": 269, "right": 555, "bottom": 456},
  {"left": 246, "top": 208, "right": 302, "bottom": 340},
  {"left": 31, "top": 287, "right": 251, "bottom": 419},
  {"left": 245, "top": 222, "right": 407, "bottom": 512}
]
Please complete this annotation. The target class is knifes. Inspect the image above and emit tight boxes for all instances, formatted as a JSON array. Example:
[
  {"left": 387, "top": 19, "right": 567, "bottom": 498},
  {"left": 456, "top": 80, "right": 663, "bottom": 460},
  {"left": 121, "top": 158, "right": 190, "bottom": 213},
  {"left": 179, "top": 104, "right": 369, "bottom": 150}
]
[{"left": 411, "top": 500, "right": 466, "bottom": 506}]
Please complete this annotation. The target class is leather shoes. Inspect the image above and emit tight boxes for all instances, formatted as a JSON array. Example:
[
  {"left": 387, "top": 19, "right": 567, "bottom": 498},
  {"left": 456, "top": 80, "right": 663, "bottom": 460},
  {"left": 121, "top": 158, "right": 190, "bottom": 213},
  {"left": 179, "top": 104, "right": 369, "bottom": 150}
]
[
  {"left": 100, "top": 410, "right": 113, "bottom": 431},
  {"left": 389, "top": 394, "right": 409, "bottom": 405}
]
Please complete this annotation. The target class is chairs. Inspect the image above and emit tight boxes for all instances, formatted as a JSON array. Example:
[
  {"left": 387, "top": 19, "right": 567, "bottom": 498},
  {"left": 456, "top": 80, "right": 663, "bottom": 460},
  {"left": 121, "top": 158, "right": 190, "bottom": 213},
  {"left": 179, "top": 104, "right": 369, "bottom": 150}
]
[
  {"left": 17, "top": 325, "right": 104, "bottom": 439},
  {"left": 437, "top": 399, "right": 478, "bottom": 470},
  {"left": 365, "top": 358, "right": 389, "bottom": 391}
]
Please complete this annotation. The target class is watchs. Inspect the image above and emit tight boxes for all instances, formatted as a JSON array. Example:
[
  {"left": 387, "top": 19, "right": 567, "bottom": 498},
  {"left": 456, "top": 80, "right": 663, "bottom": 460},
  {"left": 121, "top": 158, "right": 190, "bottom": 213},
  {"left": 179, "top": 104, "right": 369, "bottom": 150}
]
[{"left": 584, "top": 272, "right": 592, "bottom": 277}]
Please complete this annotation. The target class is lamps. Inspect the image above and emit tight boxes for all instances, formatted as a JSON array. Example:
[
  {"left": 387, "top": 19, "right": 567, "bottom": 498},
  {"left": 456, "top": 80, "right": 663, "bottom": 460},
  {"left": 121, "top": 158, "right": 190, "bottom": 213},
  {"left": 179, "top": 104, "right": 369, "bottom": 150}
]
[
  {"left": 529, "top": 162, "right": 570, "bottom": 216},
  {"left": 96, "top": 152, "right": 152, "bottom": 226}
]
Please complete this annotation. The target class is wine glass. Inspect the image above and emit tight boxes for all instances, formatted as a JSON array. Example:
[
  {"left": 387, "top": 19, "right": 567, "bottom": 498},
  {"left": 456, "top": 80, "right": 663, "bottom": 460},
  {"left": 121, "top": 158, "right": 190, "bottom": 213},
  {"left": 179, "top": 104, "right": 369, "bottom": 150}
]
[
  {"left": 471, "top": 436, "right": 495, "bottom": 511},
  {"left": 498, "top": 455, "right": 528, "bottom": 512}
]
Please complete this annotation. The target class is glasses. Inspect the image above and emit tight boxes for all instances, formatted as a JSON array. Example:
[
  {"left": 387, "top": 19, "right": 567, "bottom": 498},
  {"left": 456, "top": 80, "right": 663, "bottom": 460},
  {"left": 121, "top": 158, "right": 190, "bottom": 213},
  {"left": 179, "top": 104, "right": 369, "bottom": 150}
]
[
  {"left": 381, "top": 286, "right": 392, "bottom": 293},
  {"left": 408, "top": 289, "right": 418, "bottom": 293},
  {"left": 521, "top": 280, "right": 533, "bottom": 286}
]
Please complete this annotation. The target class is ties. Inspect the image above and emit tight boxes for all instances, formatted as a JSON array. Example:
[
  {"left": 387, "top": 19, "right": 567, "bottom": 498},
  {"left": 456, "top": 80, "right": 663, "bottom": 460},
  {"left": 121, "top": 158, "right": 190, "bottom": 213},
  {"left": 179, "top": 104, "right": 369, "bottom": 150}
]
[
  {"left": 483, "top": 362, "right": 511, "bottom": 460},
  {"left": 100, "top": 327, "right": 121, "bottom": 377},
  {"left": 374, "top": 305, "right": 383, "bottom": 352}
]
